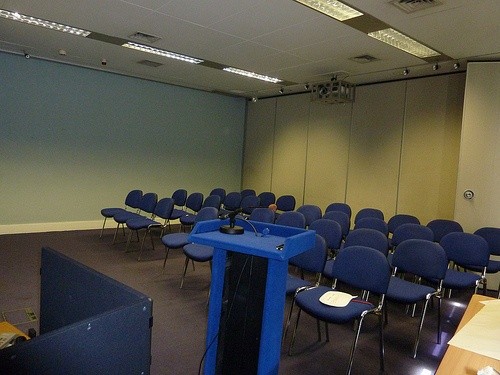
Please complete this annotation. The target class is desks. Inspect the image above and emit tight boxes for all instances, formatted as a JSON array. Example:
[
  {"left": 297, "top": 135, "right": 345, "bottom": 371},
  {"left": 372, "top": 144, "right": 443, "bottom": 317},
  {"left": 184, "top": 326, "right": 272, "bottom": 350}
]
[{"left": 434, "top": 294, "right": 500, "bottom": 375}]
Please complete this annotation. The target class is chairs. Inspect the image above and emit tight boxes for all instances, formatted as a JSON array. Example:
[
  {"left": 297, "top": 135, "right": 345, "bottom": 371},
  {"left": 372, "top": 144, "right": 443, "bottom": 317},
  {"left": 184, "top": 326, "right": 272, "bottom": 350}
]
[{"left": 99, "top": 188, "right": 500, "bottom": 375}]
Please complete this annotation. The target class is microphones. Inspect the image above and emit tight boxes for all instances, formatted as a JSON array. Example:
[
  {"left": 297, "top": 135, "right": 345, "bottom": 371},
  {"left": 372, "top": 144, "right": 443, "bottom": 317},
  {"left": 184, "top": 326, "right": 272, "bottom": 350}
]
[{"left": 220, "top": 208, "right": 242, "bottom": 220}]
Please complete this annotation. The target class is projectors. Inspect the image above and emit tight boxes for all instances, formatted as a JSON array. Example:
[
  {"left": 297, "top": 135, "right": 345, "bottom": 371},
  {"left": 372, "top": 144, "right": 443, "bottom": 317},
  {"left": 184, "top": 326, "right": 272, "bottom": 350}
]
[{"left": 319, "top": 85, "right": 350, "bottom": 99}]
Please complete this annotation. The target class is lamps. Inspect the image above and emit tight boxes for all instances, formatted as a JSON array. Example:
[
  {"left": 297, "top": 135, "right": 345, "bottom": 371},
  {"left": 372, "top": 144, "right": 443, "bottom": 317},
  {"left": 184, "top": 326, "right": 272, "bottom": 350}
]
[
  {"left": 249, "top": 97, "right": 258, "bottom": 103},
  {"left": 24, "top": 51, "right": 30, "bottom": 59}
]
